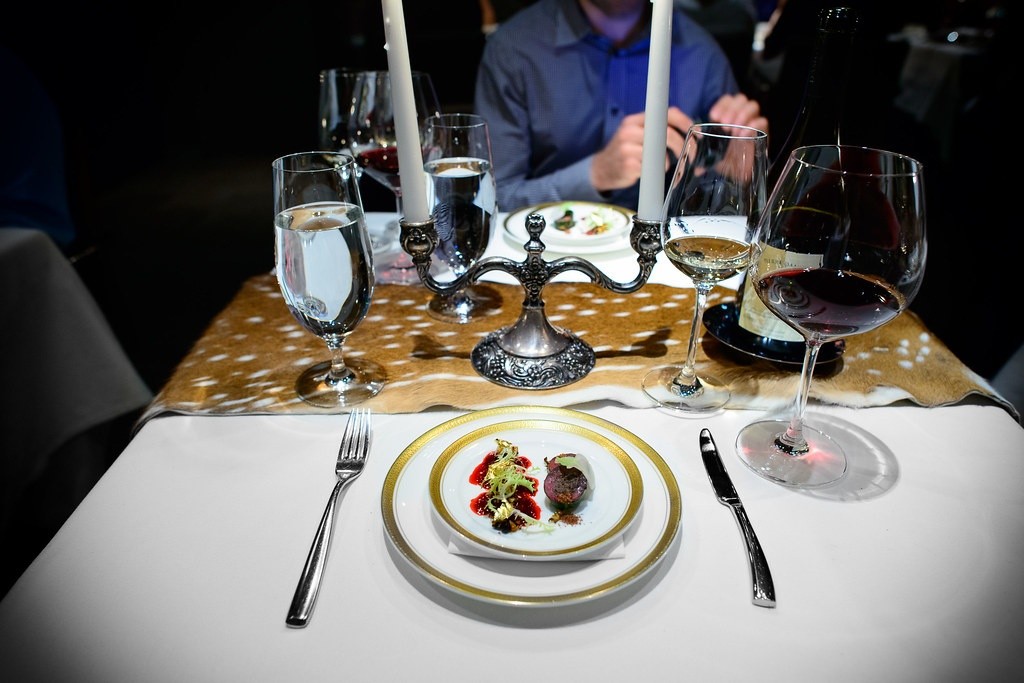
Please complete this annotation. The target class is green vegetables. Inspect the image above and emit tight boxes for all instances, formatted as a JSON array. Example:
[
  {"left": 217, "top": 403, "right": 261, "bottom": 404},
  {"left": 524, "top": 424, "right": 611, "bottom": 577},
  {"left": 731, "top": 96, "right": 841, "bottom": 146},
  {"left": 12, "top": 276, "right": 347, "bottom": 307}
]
[{"left": 486, "top": 439, "right": 554, "bottom": 532}]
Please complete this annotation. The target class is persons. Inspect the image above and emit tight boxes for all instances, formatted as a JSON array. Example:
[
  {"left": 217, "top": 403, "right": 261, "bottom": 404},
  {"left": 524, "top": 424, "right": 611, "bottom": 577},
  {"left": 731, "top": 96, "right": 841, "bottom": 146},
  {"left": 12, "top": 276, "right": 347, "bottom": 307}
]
[
  {"left": 751, "top": 0, "right": 788, "bottom": 85},
  {"left": 673, "top": 0, "right": 759, "bottom": 51},
  {"left": 469, "top": 0, "right": 772, "bottom": 217}
]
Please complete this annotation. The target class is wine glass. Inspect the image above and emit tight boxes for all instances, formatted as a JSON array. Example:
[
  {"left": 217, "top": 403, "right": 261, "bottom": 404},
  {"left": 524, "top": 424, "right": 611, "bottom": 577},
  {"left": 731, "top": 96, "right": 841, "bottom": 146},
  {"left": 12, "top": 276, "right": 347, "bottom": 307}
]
[
  {"left": 419, "top": 114, "right": 497, "bottom": 323},
  {"left": 640, "top": 123, "right": 771, "bottom": 418},
  {"left": 733, "top": 144, "right": 929, "bottom": 487},
  {"left": 272, "top": 151, "right": 387, "bottom": 409},
  {"left": 320, "top": 68, "right": 442, "bottom": 286}
]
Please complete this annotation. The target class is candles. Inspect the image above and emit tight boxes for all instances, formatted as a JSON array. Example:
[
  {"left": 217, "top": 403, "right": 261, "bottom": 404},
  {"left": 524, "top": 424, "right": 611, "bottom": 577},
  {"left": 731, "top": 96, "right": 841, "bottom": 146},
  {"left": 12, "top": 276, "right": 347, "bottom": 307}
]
[
  {"left": 630, "top": 0, "right": 674, "bottom": 224},
  {"left": 381, "top": 0, "right": 440, "bottom": 226}
]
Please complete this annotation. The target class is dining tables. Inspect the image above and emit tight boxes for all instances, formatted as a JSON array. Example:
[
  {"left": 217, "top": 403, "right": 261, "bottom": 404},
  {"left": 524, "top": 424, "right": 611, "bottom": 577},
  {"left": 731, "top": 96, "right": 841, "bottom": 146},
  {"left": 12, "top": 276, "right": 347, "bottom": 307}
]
[{"left": 0, "top": 212, "right": 1024, "bottom": 683}]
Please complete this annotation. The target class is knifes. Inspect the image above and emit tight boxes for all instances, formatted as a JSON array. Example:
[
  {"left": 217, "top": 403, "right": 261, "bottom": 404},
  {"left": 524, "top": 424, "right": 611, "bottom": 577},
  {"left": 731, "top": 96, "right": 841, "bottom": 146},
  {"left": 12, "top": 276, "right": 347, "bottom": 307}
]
[{"left": 699, "top": 427, "right": 777, "bottom": 608}]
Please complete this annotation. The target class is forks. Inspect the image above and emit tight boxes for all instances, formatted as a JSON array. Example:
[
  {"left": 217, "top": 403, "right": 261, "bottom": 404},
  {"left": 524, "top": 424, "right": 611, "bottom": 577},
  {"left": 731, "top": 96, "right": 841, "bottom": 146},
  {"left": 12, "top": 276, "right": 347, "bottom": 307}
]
[{"left": 285, "top": 406, "right": 371, "bottom": 628}]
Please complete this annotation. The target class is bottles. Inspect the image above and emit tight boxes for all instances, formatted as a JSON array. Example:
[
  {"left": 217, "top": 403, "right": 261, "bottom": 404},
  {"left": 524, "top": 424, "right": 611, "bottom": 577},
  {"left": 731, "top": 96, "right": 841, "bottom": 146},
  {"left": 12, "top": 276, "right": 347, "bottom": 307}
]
[{"left": 731, "top": 8, "right": 862, "bottom": 360}]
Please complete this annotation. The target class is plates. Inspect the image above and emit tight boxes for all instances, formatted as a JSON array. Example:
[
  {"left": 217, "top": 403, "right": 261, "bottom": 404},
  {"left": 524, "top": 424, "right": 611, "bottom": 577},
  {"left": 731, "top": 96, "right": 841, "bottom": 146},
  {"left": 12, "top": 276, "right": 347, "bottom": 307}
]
[
  {"left": 381, "top": 405, "right": 683, "bottom": 609},
  {"left": 427, "top": 418, "right": 645, "bottom": 562},
  {"left": 502, "top": 200, "right": 638, "bottom": 255},
  {"left": 697, "top": 301, "right": 845, "bottom": 365}
]
